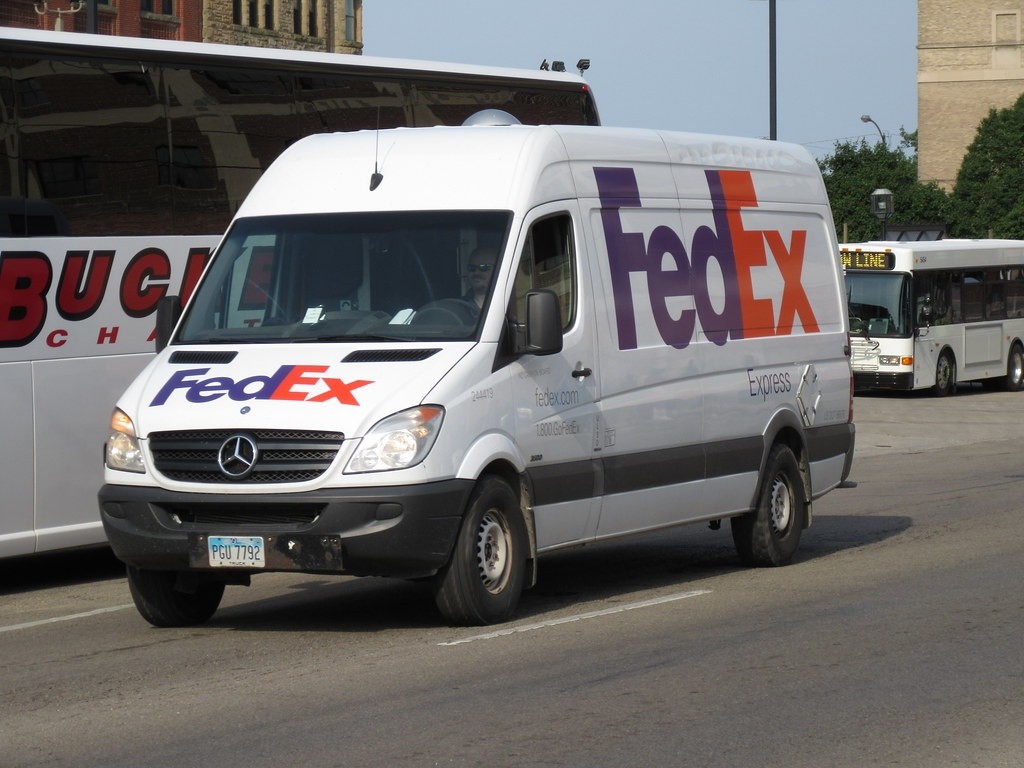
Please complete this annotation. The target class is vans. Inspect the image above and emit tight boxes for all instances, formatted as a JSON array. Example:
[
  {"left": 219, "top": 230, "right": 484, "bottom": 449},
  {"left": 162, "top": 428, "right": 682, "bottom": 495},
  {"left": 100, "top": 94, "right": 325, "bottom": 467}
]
[{"left": 98, "top": 110, "right": 855, "bottom": 626}]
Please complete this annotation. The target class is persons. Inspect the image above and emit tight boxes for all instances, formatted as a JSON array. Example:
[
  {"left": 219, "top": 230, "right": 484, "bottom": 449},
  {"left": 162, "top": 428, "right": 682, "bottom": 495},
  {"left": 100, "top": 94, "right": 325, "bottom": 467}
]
[{"left": 461, "top": 246, "right": 500, "bottom": 314}]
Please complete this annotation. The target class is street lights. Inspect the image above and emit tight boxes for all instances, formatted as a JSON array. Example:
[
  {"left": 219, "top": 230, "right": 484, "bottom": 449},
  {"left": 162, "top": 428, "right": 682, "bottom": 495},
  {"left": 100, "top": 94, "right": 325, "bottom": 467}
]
[
  {"left": 870, "top": 189, "right": 897, "bottom": 239},
  {"left": 861, "top": 115, "right": 886, "bottom": 149}
]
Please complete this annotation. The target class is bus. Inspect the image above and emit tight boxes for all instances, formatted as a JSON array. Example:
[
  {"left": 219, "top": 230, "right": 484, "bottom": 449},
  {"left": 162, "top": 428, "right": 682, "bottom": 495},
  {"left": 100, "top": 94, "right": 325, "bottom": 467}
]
[
  {"left": 838, "top": 240, "right": 1024, "bottom": 395},
  {"left": 950, "top": 279, "right": 1023, "bottom": 321},
  {"left": 0, "top": 27, "right": 602, "bottom": 560}
]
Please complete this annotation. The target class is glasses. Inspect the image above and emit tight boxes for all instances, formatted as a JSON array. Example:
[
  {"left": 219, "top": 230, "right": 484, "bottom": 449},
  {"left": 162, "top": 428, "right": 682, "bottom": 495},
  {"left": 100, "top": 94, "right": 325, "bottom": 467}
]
[{"left": 466, "top": 263, "right": 495, "bottom": 273}]
